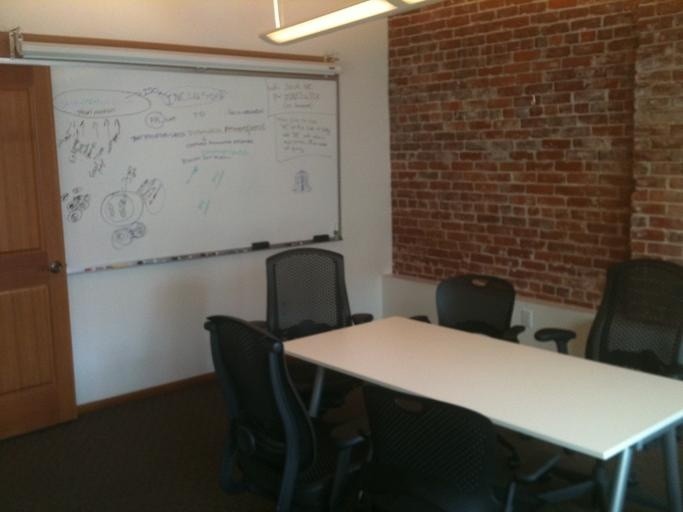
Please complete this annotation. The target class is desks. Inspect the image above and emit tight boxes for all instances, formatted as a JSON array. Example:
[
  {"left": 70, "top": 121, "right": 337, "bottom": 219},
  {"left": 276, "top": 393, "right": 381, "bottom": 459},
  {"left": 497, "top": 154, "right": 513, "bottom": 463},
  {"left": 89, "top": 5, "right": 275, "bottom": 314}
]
[{"left": 279, "top": 317, "right": 681, "bottom": 510}]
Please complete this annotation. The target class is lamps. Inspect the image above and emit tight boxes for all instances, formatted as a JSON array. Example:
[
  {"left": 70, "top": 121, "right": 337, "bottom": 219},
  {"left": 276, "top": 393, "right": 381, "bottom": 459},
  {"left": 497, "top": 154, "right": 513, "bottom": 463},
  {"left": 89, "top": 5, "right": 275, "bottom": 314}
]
[{"left": 256, "top": 0, "right": 441, "bottom": 47}]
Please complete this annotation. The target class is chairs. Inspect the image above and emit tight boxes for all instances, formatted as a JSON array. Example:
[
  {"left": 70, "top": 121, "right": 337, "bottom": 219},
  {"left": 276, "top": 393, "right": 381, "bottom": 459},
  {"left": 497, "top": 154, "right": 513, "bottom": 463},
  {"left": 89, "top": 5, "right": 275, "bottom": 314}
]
[
  {"left": 407, "top": 274, "right": 526, "bottom": 345},
  {"left": 204, "top": 314, "right": 374, "bottom": 511},
  {"left": 518, "top": 255, "right": 681, "bottom": 509},
  {"left": 263, "top": 248, "right": 374, "bottom": 438},
  {"left": 345, "top": 377, "right": 530, "bottom": 511}
]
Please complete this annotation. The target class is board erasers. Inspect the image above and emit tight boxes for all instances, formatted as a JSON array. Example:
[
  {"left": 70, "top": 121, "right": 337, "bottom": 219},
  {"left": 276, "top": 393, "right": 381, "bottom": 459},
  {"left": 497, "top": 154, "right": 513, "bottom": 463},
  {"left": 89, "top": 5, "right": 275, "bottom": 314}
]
[
  {"left": 314, "top": 235, "right": 331, "bottom": 241},
  {"left": 252, "top": 242, "right": 271, "bottom": 249}
]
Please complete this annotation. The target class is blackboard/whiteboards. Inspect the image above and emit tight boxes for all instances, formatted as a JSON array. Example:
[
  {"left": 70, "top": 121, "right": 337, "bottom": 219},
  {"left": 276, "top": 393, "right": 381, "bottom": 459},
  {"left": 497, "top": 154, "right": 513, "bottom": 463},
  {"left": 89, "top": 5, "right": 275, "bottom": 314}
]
[{"left": 24, "top": 53, "right": 342, "bottom": 274}]
